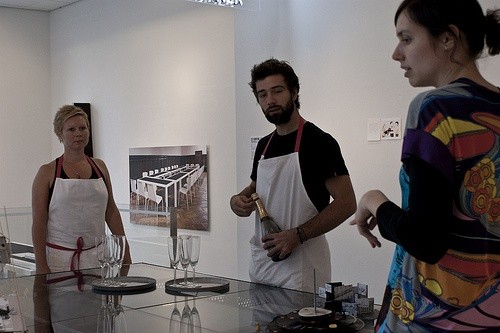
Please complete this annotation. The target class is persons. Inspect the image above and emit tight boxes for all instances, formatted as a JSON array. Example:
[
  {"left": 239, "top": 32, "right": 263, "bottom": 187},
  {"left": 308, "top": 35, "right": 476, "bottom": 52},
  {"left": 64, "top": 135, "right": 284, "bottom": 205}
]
[
  {"left": 31, "top": 104, "right": 131, "bottom": 272},
  {"left": 33, "top": 263, "right": 129, "bottom": 333},
  {"left": 350, "top": 0, "right": 500, "bottom": 333},
  {"left": 249, "top": 283, "right": 326, "bottom": 333},
  {"left": 230, "top": 56, "right": 357, "bottom": 294}
]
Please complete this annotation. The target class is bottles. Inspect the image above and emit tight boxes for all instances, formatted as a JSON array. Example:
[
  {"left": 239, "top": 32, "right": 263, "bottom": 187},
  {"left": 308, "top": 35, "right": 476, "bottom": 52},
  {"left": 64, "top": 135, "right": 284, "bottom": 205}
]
[{"left": 250, "top": 192, "right": 292, "bottom": 262}]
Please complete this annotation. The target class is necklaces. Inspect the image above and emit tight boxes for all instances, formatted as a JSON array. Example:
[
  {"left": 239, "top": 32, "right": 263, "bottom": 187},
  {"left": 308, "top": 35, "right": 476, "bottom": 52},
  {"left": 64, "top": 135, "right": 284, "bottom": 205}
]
[{"left": 75, "top": 173, "right": 81, "bottom": 178}]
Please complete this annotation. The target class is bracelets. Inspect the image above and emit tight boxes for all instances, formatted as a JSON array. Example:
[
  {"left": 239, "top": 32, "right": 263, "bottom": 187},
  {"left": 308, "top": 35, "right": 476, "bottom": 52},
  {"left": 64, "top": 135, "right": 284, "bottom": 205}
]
[{"left": 297, "top": 226, "right": 307, "bottom": 245}]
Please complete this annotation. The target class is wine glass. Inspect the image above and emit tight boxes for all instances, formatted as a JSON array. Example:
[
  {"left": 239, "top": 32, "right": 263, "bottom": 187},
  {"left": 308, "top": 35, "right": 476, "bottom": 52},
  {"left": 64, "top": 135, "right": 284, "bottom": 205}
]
[
  {"left": 169, "top": 295, "right": 201, "bottom": 333},
  {"left": 167, "top": 234, "right": 202, "bottom": 289},
  {"left": 96, "top": 294, "right": 127, "bottom": 333},
  {"left": 95, "top": 234, "right": 127, "bottom": 288}
]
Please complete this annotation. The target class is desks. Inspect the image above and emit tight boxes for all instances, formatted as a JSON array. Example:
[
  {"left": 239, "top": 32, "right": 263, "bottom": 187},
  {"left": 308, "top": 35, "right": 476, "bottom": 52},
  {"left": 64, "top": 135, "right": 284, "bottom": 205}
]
[
  {"left": 166, "top": 171, "right": 188, "bottom": 206},
  {"left": 168, "top": 168, "right": 181, "bottom": 175},
  {"left": 0, "top": 261, "right": 384, "bottom": 333},
  {"left": 184, "top": 165, "right": 200, "bottom": 169},
  {"left": 178, "top": 165, "right": 186, "bottom": 170},
  {"left": 140, "top": 176, "right": 177, "bottom": 216},
  {"left": 183, "top": 168, "right": 196, "bottom": 174},
  {"left": 149, "top": 171, "right": 168, "bottom": 178}
]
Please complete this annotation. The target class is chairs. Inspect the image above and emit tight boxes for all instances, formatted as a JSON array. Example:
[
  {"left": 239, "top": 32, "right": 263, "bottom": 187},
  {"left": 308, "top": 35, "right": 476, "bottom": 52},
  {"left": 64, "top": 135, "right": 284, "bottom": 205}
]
[{"left": 130, "top": 163, "right": 205, "bottom": 212}]
